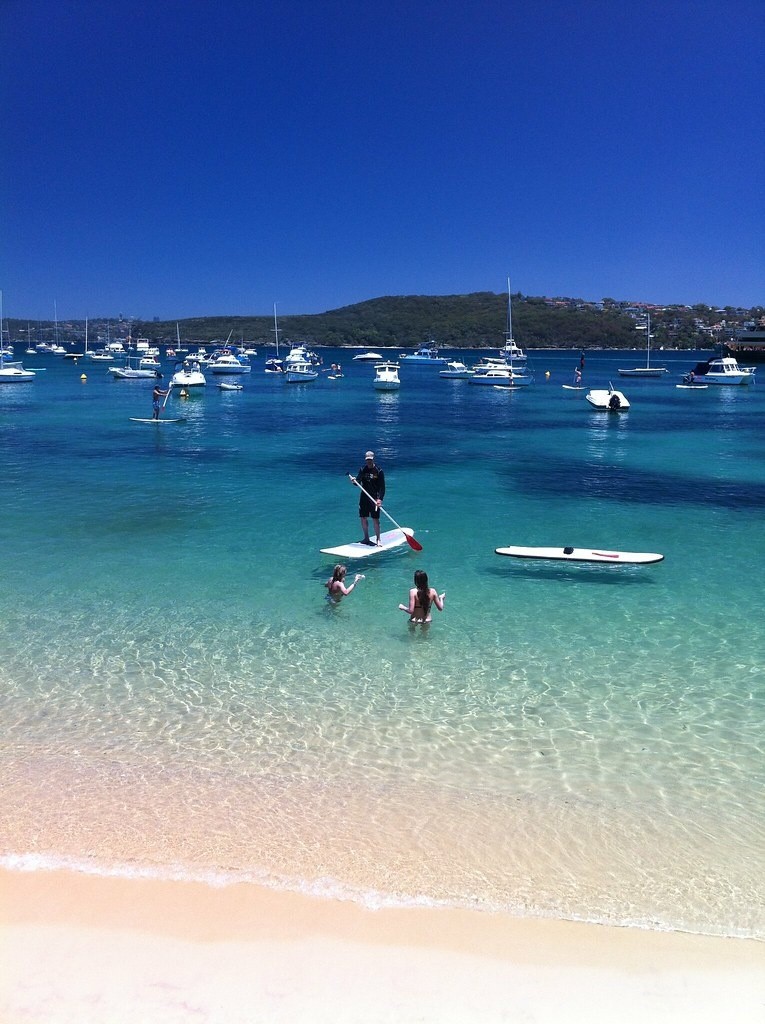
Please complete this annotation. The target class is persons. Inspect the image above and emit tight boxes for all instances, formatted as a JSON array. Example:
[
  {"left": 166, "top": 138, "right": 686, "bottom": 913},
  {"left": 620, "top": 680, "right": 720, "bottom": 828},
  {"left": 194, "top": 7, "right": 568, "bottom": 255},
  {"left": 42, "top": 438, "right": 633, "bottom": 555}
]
[
  {"left": 350, "top": 451, "right": 385, "bottom": 547},
  {"left": 324, "top": 565, "right": 361, "bottom": 595},
  {"left": 398, "top": 570, "right": 446, "bottom": 624},
  {"left": 691, "top": 370, "right": 694, "bottom": 386},
  {"left": 575, "top": 369, "right": 581, "bottom": 387},
  {"left": 331, "top": 362, "right": 337, "bottom": 377},
  {"left": 152, "top": 384, "right": 168, "bottom": 419},
  {"left": 337, "top": 363, "right": 342, "bottom": 375}
]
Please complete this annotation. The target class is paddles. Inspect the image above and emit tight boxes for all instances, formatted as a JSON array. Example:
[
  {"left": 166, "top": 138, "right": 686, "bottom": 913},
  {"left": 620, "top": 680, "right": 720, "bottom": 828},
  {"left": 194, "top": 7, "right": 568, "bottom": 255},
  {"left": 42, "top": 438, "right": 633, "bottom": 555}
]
[
  {"left": 345, "top": 471, "right": 423, "bottom": 551},
  {"left": 161, "top": 389, "right": 172, "bottom": 413}
]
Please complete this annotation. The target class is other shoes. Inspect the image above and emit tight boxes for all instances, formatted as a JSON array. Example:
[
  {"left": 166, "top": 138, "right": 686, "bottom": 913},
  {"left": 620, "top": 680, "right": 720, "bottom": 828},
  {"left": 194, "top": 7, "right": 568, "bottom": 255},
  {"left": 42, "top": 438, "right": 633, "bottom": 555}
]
[
  {"left": 362, "top": 537, "right": 370, "bottom": 544},
  {"left": 377, "top": 540, "right": 381, "bottom": 548}
]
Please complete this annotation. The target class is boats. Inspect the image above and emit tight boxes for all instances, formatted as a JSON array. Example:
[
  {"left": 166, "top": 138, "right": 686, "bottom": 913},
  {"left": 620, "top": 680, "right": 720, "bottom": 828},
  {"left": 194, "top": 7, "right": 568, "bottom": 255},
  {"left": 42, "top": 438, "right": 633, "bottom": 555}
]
[
  {"left": 71, "top": 341, "right": 77, "bottom": 346},
  {"left": 439, "top": 361, "right": 475, "bottom": 379},
  {"left": 286, "top": 344, "right": 324, "bottom": 385},
  {"left": 373, "top": 359, "right": 401, "bottom": 389},
  {"left": 586, "top": 380, "right": 630, "bottom": 413},
  {"left": 399, "top": 340, "right": 452, "bottom": 364},
  {"left": 165, "top": 342, "right": 259, "bottom": 391},
  {"left": 91, "top": 337, "right": 163, "bottom": 378},
  {"left": 471, "top": 338, "right": 528, "bottom": 376},
  {"left": 353, "top": 352, "right": 384, "bottom": 361},
  {"left": 682, "top": 357, "right": 756, "bottom": 385},
  {"left": 64, "top": 352, "right": 84, "bottom": 359}
]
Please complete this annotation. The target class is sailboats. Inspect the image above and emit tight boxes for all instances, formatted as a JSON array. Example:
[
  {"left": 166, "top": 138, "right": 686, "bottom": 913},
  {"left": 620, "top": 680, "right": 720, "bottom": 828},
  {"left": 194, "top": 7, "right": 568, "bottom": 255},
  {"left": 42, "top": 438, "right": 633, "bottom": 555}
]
[
  {"left": 469, "top": 278, "right": 536, "bottom": 386},
  {"left": 85, "top": 317, "right": 95, "bottom": 355},
  {"left": 618, "top": 314, "right": 669, "bottom": 377},
  {"left": 264, "top": 301, "right": 284, "bottom": 370},
  {"left": 0, "top": 289, "right": 37, "bottom": 382},
  {"left": 25, "top": 301, "right": 66, "bottom": 357},
  {"left": 174, "top": 323, "right": 188, "bottom": 353}
]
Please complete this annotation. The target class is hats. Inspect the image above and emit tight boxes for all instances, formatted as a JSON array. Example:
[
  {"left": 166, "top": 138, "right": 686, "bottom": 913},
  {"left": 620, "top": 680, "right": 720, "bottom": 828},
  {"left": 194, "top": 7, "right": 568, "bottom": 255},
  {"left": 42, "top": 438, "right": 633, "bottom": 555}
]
[
  {"left": 155, "top": 385, "right": 161, "bottom": 389},
  {"left": 365, "top": 450, "right": 375, "bottom": 460}
]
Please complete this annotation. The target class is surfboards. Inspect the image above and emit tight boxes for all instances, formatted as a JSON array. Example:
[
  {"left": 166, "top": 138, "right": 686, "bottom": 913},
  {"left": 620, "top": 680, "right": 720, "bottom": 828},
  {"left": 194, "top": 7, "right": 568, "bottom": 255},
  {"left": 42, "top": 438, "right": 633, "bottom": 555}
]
[
  {"left": 676, "top": 384, "right": 708, "bottom": 389},
  {"left": 129, "top": 417, "right": 178, "bottom": 422},
  {"left": 562, "top": 385, "right": 589, "bottom": 390},
  {"left": 494, "top": 546, "right": 665, "bottom": 564},
  {"left": 319, "top": 527, "right": 414, "bottom": 559}
]
